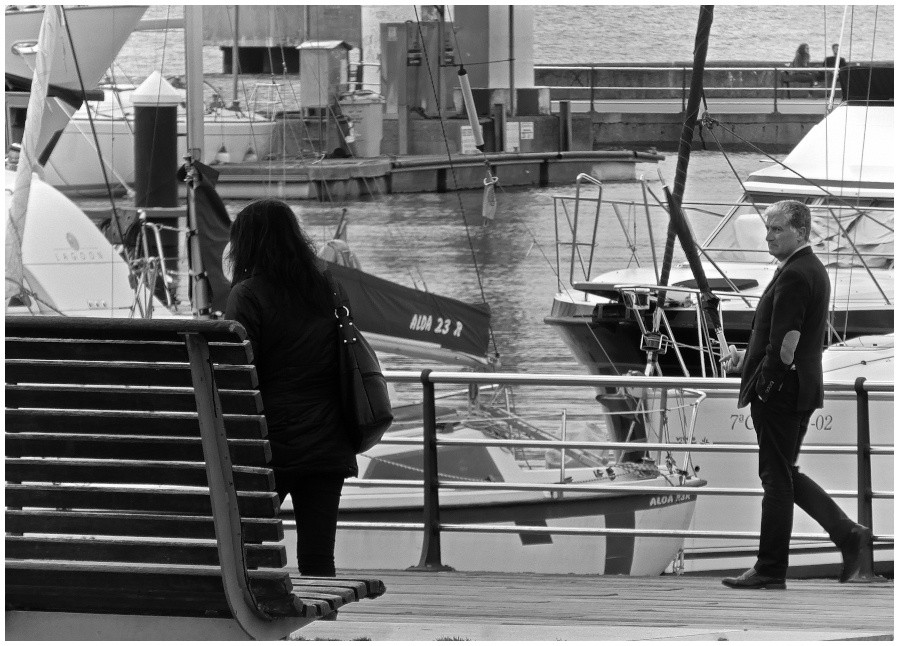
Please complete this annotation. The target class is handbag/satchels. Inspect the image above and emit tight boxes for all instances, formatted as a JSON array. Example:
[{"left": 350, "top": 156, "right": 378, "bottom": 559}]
[{"left": 323, "top": 268, "right": 394, "bottom": 454}]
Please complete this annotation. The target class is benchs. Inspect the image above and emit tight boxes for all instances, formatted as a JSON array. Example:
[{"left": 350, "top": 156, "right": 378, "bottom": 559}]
[{"left": 5, "top": 315, "right": 388, "bottom": 640}]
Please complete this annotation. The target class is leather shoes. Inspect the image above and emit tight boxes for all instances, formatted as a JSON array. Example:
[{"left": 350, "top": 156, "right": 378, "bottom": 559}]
[
  {"left": 721, "top": 565, "right": 786, "bottom": 589},
  {"left": 839, "top": 524, "right": 872, "bottom": 583}
]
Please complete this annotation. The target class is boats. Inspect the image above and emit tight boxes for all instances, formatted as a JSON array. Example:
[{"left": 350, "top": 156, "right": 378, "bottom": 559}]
[
  {"left": 5, "top": 5, "right": 707, "bottom": 576},
  {"left": 543, "top": 5, "right": 894, "bottom": 578}
]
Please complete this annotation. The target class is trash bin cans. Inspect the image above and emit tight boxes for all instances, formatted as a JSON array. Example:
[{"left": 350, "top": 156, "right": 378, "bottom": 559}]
[{"left": 338, "top": 89, "right": 385, "bottom": 157}]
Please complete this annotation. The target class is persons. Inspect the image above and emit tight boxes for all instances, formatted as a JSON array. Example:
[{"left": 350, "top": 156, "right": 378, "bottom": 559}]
[
  {"left": 225, "top": 199, "right": 358, "bottom": 620},
  {"left": 721, "top": 200, "right": 868, "bottom": 589},
  {"left": 821, "top": 44, "right": 847, "bottom": 81},
  {"left": 784, "top": 43, "right": 817, "bottom": 99}
]
